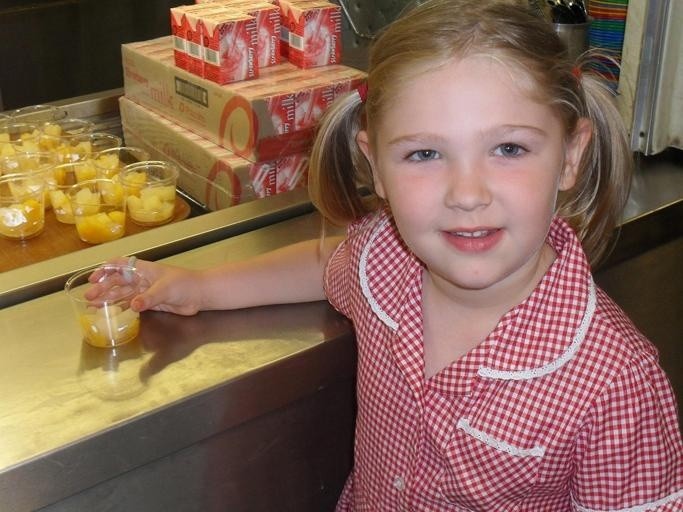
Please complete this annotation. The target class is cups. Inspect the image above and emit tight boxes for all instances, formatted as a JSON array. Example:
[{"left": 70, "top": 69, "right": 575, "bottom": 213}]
[
  {"left": 0, "top": 105, "right": 179, "bottom": 246},
  {"left": 64, "top": 265, "right": 144, "bottom": 347},
  {"left": 553, "top": 21, "right": 589, "bottom": 66}
]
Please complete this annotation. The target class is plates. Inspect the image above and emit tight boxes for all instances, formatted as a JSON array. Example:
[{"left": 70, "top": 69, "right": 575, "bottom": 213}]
[{"left": 585, "top": 0, "right": 628, "bottom": 90}]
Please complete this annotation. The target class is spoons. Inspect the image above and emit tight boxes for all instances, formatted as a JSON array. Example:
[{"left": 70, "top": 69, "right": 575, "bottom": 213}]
[{"left": 549, "top": 1, "right": 587, "bottom": 23}]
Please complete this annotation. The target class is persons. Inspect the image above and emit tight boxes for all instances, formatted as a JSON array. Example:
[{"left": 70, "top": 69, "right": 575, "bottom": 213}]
[{"left": 83, "top": 0, "right": 683, "bottom": 512}]
